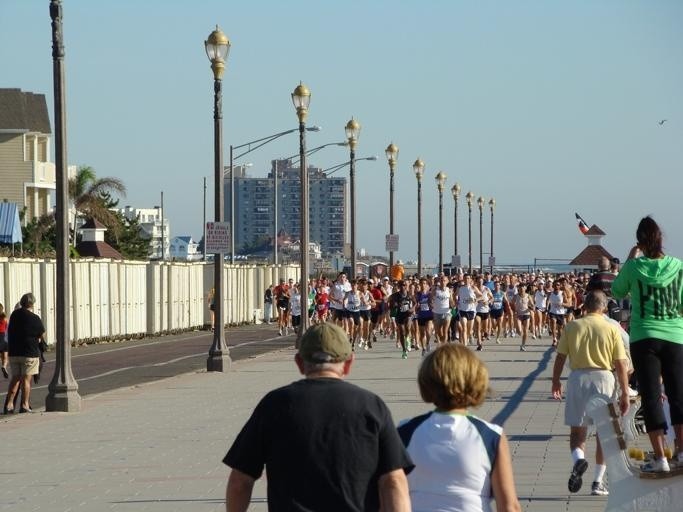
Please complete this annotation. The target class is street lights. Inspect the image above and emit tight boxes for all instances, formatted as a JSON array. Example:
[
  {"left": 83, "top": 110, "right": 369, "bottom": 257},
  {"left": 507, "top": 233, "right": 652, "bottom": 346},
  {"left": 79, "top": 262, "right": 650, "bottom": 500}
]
[
  {"left": 413, "top": 156, "right": 426, "bottom": 278},
  {"left": 449, "top": 181, "right": 461, "bottom": 256},
  {"left": 288, "top": 79, "right": 313, "bottom": 346},
  {"left": 222, "top": 124, "right": 379, "bottom": 263},
  {"left": 384, "top": 140, "right": 399, "bottom": 276},
  {"left": 435, "top": 170, "right": 446, "bottom": 273},
  {"left": 477, "top": 196, "right": 484, "bottom": 274},
  {"left": 201, "top": 23, "right": 232, "bottom": 373},
  {"left": 464, "top": 191, "right": 474, "bottom": 273},
  {"left": 344, "top": 116, "right": 361, "bottom": 285},
  {"left": 488, "top": 198, "right": 495, "bottom": 276}
]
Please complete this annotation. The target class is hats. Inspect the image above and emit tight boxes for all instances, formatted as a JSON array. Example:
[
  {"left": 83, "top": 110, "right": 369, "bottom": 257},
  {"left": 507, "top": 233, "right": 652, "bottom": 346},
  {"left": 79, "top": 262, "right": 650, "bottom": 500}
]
[{"left": 297, "top": 322, "right": 356, "bottom": 363}]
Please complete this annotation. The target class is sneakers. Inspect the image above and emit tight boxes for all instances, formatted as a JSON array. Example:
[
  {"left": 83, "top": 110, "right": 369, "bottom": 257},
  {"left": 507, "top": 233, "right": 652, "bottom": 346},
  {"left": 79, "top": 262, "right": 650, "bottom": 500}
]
[
  {"left": 360, "top": 326, "right": 581, "bottom": 360},
  {"left": 592, "top": 479, "right": 612, "bottom": 499},
  {"left": 568, "top": 459, "right": 590, "bottom": 494},
  {"left": 638, "top": 449, "right": 683, "bottom": 478}
]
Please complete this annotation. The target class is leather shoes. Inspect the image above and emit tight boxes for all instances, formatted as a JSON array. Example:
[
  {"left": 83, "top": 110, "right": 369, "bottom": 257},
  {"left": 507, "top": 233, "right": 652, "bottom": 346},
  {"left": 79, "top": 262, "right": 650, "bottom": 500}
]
[{"left": 1, "top": 402, "right": 38, "bottom": 417}]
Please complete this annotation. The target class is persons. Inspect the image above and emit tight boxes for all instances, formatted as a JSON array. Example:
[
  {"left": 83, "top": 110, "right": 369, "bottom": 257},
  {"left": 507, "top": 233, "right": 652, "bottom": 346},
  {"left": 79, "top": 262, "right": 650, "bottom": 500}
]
[
  {"left": 0, "top": 293, "right": 44, "bottom": 413},
  {"left": 221, "top": 323, "right": 416, "bottom": 512},
  {"left": 551, "top": 216, "right": 683, "bottom": 495},
  {"left": 396, "top": 344, "right": 523, "bottom": 512},
  {"left": 265, "top": 260, "right": 570, "bottom": 359},
  {"left": 207, "top": 288, "right": 215, "bottom": 331}
]
